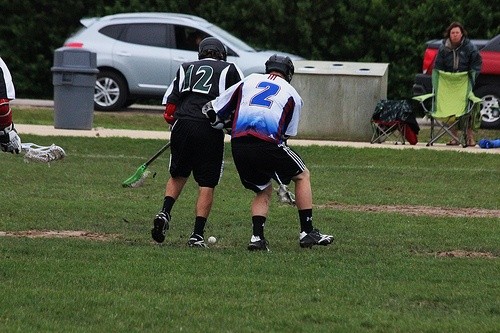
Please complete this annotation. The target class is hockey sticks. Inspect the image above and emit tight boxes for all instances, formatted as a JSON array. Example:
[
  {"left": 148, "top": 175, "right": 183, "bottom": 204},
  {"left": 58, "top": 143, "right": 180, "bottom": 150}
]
[
  {"left": 21, "top": 143, "right": 66, "bottom": 163},
  {"left": 122, "top": 141, "right": 171, "bottom": 189},
  {"left": 272, "top": 171, "right": 296, "bottom": 207}
]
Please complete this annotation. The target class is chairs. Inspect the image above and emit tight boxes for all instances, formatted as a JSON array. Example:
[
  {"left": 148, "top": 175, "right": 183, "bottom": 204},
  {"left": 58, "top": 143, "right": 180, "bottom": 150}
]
[
  {"left": 370, "top": 99, "right": 420, "bottom": 145},
  {"left": 410, "top": 68, "right": 483, "bottom": 148}
]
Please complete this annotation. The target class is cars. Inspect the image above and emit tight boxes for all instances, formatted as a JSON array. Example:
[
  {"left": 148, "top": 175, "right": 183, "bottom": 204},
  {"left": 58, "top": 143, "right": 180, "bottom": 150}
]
[{"left": 412, "top": 35, "right": 499, "bottom": 130}]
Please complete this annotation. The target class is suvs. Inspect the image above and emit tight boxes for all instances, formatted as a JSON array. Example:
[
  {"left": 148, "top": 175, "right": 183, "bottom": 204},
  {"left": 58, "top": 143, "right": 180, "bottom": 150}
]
[{"left": 62, "top": 14, "right": 310, "bottom": 113}]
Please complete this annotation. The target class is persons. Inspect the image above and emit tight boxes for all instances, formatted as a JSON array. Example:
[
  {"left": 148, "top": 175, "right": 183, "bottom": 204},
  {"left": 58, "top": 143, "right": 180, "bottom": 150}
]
[
  {"left": 189, "top": 31, "right": 207, "bottom": 51},
  {"left": 434, "top": 22, "right": 483, "bottom": 146},
  {"left": 0, "top": 58, "right": 21, "bottom": 154},
  {"left": 150, "top": 37, "right": 245, "bottom": 248},
  {"left": 202, "top": 54, "right": 336, "bottom": 250}
]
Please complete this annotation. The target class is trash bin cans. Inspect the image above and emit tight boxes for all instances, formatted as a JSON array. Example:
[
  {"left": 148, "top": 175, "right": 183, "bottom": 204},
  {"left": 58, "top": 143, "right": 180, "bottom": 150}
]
[{"left": 50, "top": 46, "right": 100, "bottom": 130}]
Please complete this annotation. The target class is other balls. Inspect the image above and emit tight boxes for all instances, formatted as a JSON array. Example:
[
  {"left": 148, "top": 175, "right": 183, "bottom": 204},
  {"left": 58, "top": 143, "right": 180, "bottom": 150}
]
[{"left": 208, "top": 236, "right": 216, "bottom": 243}]
